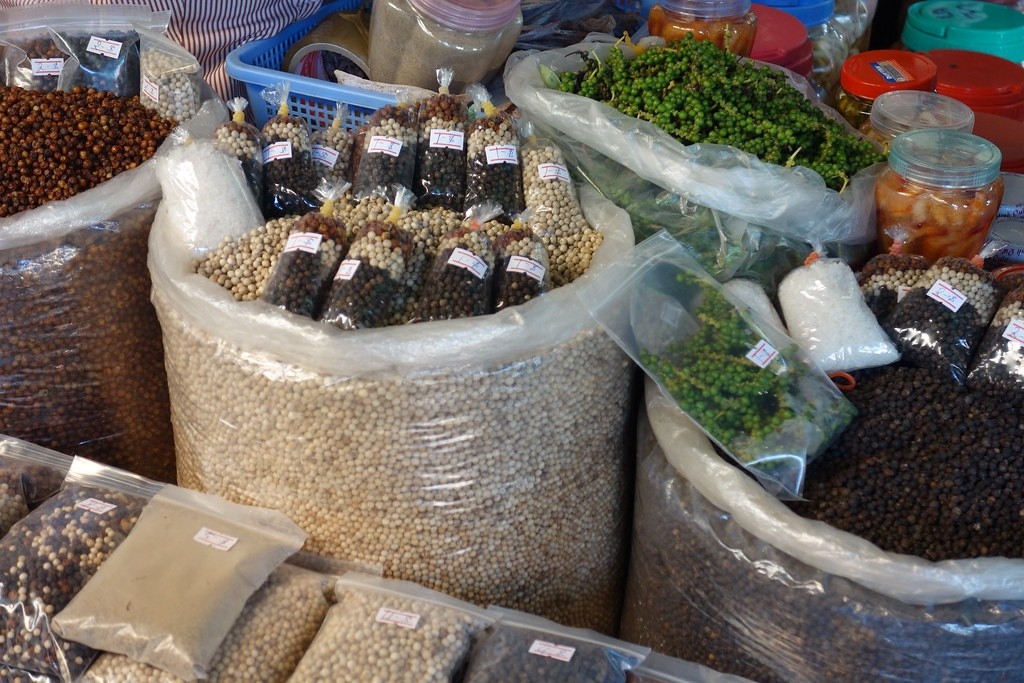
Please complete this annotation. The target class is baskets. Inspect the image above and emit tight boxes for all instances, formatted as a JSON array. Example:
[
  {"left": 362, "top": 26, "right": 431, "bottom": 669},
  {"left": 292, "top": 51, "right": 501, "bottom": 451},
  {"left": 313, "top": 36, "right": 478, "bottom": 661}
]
[{"left": 227, "top": 0, "right": 400, "bottom": 137}]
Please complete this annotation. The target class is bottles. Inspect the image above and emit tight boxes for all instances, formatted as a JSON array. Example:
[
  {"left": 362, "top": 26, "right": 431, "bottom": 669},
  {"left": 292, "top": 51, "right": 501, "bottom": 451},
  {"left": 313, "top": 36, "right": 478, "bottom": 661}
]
[
  {"left": 834, "top": 50, "right": 1005, "bottom": 265},
  {"left": 367, "top": 0, "right": 523, "bottom": 95},
  {"left": 647, "top": 0, "right": 758, "bottom": 58}
]
